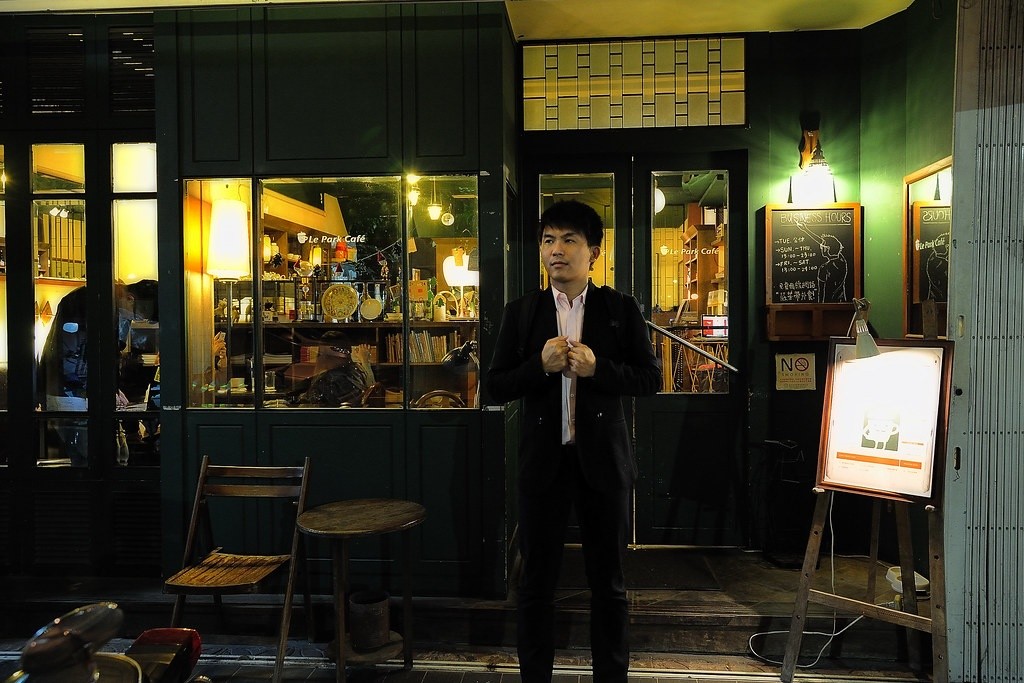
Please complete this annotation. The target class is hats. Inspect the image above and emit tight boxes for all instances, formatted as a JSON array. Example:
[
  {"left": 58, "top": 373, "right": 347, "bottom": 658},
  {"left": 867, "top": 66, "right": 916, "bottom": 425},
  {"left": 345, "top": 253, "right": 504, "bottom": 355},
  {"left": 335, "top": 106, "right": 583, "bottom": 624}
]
[{"left": 313, "top": 330, "right": 351, "bottom": 347}]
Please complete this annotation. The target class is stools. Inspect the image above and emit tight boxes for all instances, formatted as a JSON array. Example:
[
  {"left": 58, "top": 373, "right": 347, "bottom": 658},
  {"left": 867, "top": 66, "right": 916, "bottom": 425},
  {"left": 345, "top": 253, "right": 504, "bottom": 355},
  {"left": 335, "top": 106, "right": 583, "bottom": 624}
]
[{"left": 698, "top": 363, "right": 722, "bottom": 391}]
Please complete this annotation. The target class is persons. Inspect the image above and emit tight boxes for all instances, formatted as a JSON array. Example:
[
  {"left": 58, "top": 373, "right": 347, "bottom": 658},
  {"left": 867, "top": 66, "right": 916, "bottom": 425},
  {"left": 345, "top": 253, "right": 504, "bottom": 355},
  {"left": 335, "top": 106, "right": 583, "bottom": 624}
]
[
  {"left": 136, "top": 328, "right": 226, "bottom": 463},
  {"left": 45, "top": 280, "right": 160, "bottom": 467},
  {"left": 291, "top": 330, "right": 369, "bottom": 408},
  {"left": 479, "top": 198, "right": 663, "bottom": 683}
]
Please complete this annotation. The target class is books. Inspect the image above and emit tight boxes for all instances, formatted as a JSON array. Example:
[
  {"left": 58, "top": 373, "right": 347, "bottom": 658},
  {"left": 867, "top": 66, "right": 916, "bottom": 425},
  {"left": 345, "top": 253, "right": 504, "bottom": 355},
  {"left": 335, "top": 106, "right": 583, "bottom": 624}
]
[
  {"left": 133, "top": 353, "right": 160, "bottom": 366},
  {"left": 385, "top": 330, "right": 447, "bottom": 364}
]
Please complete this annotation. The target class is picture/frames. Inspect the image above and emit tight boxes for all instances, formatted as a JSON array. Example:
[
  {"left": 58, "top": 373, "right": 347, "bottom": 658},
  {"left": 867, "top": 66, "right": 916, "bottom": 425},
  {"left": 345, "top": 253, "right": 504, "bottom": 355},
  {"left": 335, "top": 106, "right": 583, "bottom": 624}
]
[{"left": 901, "top": 155, "right": 952, "bottom": 340}]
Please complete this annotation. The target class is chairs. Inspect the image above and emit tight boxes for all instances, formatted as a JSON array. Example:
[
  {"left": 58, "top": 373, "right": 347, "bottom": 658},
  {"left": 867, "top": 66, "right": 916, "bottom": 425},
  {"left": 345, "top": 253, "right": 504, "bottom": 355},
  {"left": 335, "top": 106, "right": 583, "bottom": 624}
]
[
  {"left": 165, "top": 455, "right": 312, "bottom": 683},
  {"left": 359, "top": 381, "right": 385, "bottom": 408},
  {"left": 415, "top": 390, "right": 467, "bottom": 408}
]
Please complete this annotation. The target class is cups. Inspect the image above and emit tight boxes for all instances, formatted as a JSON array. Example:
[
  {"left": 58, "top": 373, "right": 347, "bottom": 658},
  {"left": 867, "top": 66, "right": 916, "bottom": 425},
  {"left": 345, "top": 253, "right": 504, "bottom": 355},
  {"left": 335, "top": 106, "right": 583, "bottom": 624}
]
[
  {"left": 265, "top": 371, "right": 276, "bottom": 389},
  {"left": 433, "top": 304, "right": 446, "bottom": 321}
]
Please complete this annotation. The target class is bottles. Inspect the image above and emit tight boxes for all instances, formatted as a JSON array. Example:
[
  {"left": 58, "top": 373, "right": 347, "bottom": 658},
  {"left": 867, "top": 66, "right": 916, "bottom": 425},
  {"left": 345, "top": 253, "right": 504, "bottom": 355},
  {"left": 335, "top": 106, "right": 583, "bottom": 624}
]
[
  {"left": 360, "top": 282, "right": 371, "bottom": 308},
  {"left": 424, "top": 278, "right": 434, "bottom": 320}
]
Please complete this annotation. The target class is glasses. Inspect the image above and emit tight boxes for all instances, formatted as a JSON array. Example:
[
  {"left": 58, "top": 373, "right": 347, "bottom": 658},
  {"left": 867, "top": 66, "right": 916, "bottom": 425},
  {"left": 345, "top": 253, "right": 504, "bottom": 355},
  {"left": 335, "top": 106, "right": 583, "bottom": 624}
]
[{"left": 133, "top": 298, "right": 143, "bottom": 321}]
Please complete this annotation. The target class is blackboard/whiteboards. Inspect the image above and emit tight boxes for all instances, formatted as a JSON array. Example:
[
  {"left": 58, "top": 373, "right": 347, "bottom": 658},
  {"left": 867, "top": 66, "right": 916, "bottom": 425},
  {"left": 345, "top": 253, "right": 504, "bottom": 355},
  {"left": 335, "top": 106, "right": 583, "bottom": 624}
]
[
  {"left": 766, "top": 203, "right": 861, "bottom": 309},
  {"left": 912, "top": 199, "right": 949, "bottom": 303}
]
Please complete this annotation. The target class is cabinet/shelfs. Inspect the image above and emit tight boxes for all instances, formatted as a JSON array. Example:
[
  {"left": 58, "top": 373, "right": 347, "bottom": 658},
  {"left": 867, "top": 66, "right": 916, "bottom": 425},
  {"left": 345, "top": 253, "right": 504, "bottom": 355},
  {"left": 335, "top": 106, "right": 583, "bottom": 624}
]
[
  {"left": 0, "top": 237, "right": 51, "bottom": 277},
  {"left": 214, "top": 320, "right": 480, "bottom": 401},
  {"left": 247, "top": 211, "right": 338, "bottom": 279},
  {"left": 710, "top": 224, "right": 728, "bottom": 290},
  {"left": 680, "top": 224, "right": 727, "bottom": 391}
]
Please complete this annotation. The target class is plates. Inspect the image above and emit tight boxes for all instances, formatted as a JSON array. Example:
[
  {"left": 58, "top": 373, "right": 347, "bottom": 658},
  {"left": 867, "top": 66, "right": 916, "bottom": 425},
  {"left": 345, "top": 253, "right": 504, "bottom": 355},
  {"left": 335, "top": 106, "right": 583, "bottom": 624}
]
[
  {"left": 360, "top": 298, "right": 383, "bottom": 320},
  {"left": 321, "top": 284, "right": 359, "bottom": 320}
]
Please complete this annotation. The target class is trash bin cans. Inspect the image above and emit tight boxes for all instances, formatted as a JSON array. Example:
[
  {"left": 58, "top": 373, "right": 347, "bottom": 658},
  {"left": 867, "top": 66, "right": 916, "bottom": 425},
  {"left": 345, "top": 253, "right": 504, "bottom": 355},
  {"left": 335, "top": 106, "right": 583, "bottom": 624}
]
[{"left": 348, "top": 591, "right": 390, "bottom": 652}]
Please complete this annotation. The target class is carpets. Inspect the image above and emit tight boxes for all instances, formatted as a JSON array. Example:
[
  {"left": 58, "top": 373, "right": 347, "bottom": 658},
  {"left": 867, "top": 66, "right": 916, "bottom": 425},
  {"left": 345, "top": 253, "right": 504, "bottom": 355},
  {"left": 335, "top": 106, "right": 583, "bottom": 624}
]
[{"left": 517, "top": 548, "right": 728, "bottom": 591}]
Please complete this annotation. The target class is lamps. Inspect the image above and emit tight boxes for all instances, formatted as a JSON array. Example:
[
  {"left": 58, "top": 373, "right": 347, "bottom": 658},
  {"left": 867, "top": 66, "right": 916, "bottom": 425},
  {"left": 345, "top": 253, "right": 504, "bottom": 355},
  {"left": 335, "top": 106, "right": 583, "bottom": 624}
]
[
  {"left": 442, "top": 255, "right": 479, "bottom": 320},
  {"left": 263, "top": 234, "right": 272, "bottom": 264},
  {"left": 206, "top": 198, "right": 250, "bottom": 405},
  {"left": 309, "top": 245, "right": 321, "bottom": 267},
  {"left": 440, "top": 340, "right": 480, "bottom": 376},
  {"left": 790, "top": 128, "right": 837, "bottom": 204},
  {"left": 49, "top": 206, "right": 61, "bottom": 216},
  {"left": 659, "top": 213, "right": 668, "bottom": 256},
  {"left": 427, "top": 180, "right": 443, "bottom": 220},
  {"left": 655, "top": 179, "right": 665, "bottom": 214},
  {"left": 59, "top": 208, "right": 70, "bottom": 218}
]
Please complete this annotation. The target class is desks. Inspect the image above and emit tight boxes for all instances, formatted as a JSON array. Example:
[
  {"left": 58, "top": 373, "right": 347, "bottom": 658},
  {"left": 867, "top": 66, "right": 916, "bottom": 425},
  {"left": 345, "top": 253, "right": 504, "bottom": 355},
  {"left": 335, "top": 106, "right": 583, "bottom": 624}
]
[
  {"left": 214, "top": 381, "right": 311, "bottom": 408},
  {"left": 296, "top": 498, "right": 428, "bottom": 683},
  {"left": 116, "top": 401, "right": 160, "bottom": 451},
  {"left": 689, "top": 337, "right": 727, "bottom": 393}
]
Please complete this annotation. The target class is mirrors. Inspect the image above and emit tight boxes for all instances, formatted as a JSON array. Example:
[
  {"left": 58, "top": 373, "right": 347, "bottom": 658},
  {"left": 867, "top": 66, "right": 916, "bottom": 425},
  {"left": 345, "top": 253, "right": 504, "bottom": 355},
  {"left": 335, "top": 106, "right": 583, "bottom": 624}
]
[{"left": 0, "top": 154, "right": 87, "bottom": 287}]
